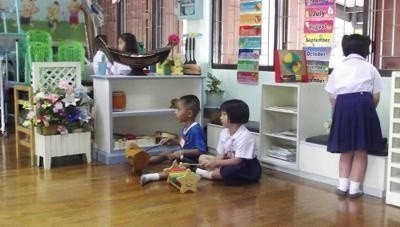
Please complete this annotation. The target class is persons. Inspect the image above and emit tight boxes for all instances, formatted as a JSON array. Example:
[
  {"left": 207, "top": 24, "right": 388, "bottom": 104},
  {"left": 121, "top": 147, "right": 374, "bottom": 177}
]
[
  {"left": 112, "top": 33, "right": 148, "bottom": 76},
  {"left": 68, "top": 0, "right": 81, "bottom": 30},
  {"left": 46, "top": 1, "right": 60, "bottom": 34},
  {"left": 11, "top": 0, "right": 41, "bottom": 28},
  {"left": 91, "top": 34, "right": 110, "bottom": 76},
  {"left": 323, "top": 32, "right": 384, "bottom": 198},
  {"left": 196, "top": 98, "right": 262, "bottom": 183},
  {"left": 138, "top": 95, "right": 207, "bottom": 187}
]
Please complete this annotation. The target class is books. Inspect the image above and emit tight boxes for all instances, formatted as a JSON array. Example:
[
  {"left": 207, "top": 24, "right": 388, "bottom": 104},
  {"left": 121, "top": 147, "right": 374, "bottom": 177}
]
[{"left": 267, "top": 142, "right": 297, "bottom": 162}]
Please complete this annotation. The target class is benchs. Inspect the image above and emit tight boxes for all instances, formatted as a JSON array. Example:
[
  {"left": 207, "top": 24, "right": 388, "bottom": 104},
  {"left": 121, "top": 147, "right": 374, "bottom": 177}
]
[
  {"left": 299, "top": 134, "right": 388, "bottom": 198},
  {"left": 207, "top": 121, "right": 259, "bottom": 160}
]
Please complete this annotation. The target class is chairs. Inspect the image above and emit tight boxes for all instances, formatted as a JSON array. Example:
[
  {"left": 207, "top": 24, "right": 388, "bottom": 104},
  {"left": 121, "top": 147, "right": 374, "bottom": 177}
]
[
  {"left": 0, "top": 0, "right": 93, "bottom": 137},
  {"left": 32, "top": 61, "right": 92, "bottom": 169}
]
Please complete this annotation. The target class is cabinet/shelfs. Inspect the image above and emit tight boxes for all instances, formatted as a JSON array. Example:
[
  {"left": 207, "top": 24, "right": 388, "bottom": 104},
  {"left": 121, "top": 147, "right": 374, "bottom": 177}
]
[
  {"left": 92, "top": 74, "right": 206, "bottom": 166},
  {"left": 261, "top": 83, "right": 332, "bottom": 178},
  {"left": 385, "top": 71, "right": 400, "bottom": 206},
  {"left": 14, "top": 85, "right": 35, "bottom": 167}
]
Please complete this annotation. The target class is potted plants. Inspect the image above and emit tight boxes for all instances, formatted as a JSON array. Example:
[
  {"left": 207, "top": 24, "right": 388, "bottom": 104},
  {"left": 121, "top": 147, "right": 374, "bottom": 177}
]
[{"left": 204, "top": 72, "right": 225, "bottom": 109}]
